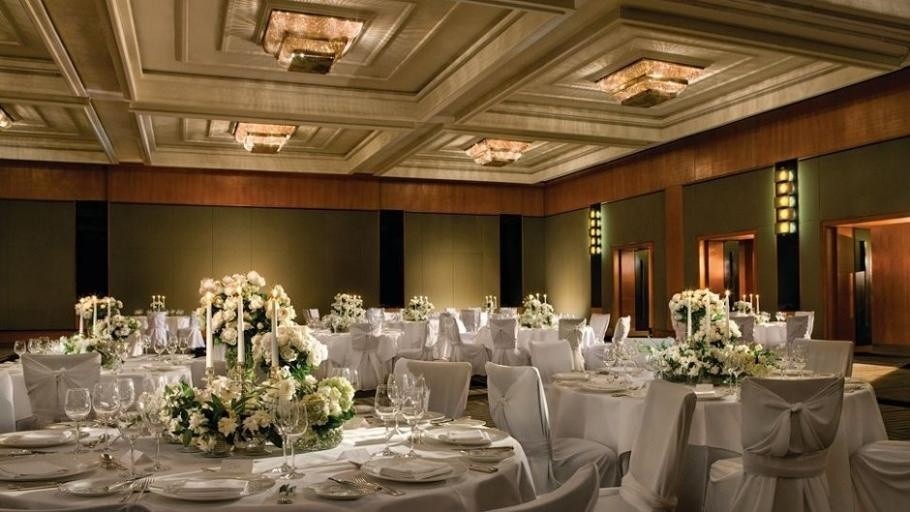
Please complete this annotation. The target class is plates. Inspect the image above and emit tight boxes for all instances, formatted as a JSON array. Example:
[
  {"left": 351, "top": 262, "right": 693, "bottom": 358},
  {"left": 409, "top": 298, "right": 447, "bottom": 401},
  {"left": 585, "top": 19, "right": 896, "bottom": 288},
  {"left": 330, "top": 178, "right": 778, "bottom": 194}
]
[
  {"left": 425, "top": 424, "right": 510, "bottom": 446},
  {"left": 60, "top": 476, "right": 137, "bottom": 497},
  {"left": 1, "top": 453, "right": 103, "bottom": 483},
  {"left": 362, "top": 455, "right": 467, "bottom": 480},
  {"left": 0, "top": 429, "right": 75, "bottom": 449},
  {"left": 550, "top": 337, "right": 869, "bottom": 403},
  {"left": 148, "top": 470, "right": 276, "bottom": 501},
  {"left": 309, "top": 481, "right": 366, "bottom": 501}
]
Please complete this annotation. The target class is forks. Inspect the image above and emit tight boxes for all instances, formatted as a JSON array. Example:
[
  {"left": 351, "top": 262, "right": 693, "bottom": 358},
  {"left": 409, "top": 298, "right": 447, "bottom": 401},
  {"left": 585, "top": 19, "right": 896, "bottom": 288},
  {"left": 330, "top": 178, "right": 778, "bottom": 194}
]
[{"left": 132, "top": 478, "right": 152, "bottom": 504}]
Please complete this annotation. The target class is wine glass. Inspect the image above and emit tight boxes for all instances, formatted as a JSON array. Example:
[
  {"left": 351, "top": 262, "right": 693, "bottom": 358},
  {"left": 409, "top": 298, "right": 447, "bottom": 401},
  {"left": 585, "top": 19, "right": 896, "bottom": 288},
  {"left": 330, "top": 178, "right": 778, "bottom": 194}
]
[
  {"left": 63, "top": 388, "right": 92, "bottom": 453},
  {"left": 13, "top": 333, "right": 190, "bottom": 371},
  {"left": 92, "top": 382, "right": 122, "bottom": 453},
  {"left": 116, "top": 401, "right": 143, "bottom": 479},
  {"left": 401, "top": 390, "right": 424, "bottom": 460},
  {"left": 403, "top": 375, "right": 426, "bottom": 445},
  {"left": 142, "top": 395, "right": 171, "bottom": 476},
  {"left": 492, "top": 312, "right": 575, "bottom": 330},
  {"left": 270, "top": 395, "right": 299, "bottom": 476},
  {"left": 759, "top": 310, "right": 789, "bottom": 324},
  {"left": 386, "top": 373, "right": 410, "bottom": 434},
  {"left": 113, "top": 377, "right": 135, "bottom": 435},
  {"left": 281, "top": 403, "right": 306, "bottom": 480},
  {"left": 374, "top": 383, "right": 399, "bottom": 457}
]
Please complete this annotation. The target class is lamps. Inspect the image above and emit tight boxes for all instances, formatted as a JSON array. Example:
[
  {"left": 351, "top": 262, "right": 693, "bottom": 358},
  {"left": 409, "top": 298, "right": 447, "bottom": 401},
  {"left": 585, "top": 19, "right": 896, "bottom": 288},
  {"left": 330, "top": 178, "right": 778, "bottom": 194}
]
[
  {"left": 584, "top": 48, "right": 717, "bottom": 111},
  {"left": 456, "top": 134, "right": 537, "bottom": 168},
  {"left": 249, "top": 1, "right": 379, "bottom": 74},
  {"left": 233, "top": 122, "right": 298, "bottom": 154}
]
